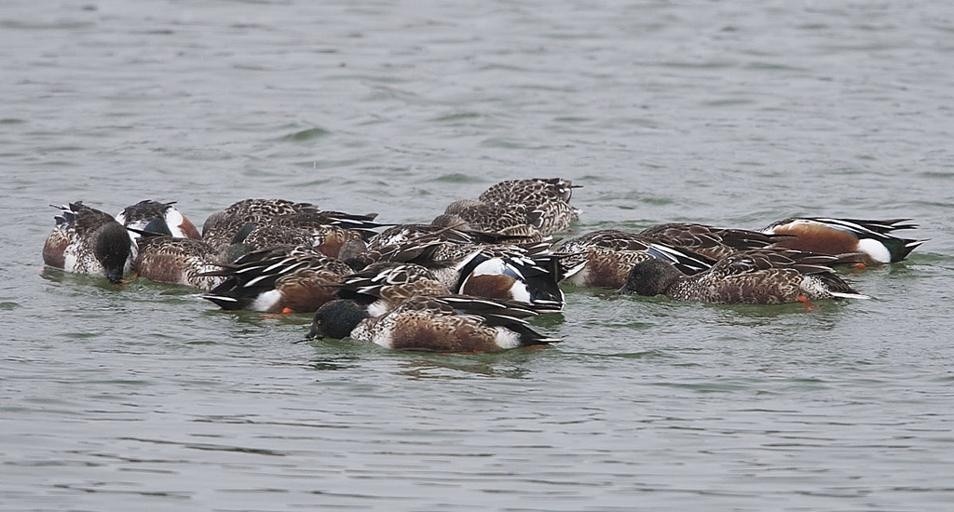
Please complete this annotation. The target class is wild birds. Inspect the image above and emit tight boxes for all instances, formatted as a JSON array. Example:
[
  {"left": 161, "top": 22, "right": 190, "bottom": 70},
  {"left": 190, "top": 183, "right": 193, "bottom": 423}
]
[
  {"left": 39, "top": 175, "right": 586, "bottom": 351},
  {"left": 554, "top": 215, "right": 927, "bottom": 313}
]
